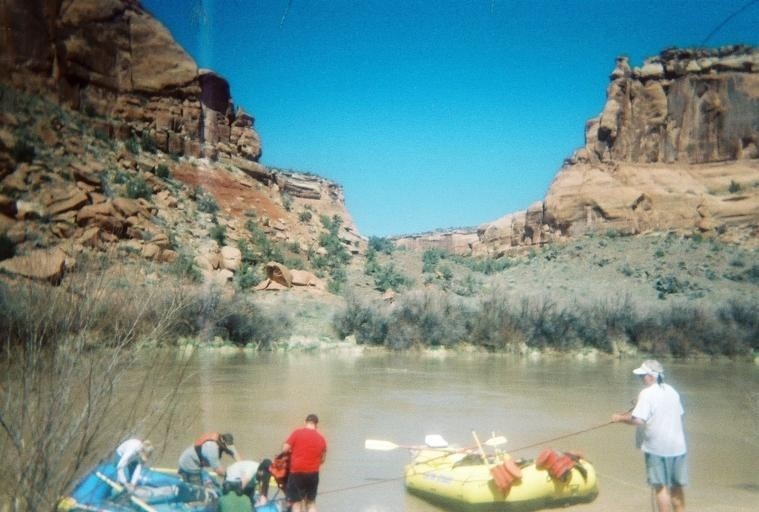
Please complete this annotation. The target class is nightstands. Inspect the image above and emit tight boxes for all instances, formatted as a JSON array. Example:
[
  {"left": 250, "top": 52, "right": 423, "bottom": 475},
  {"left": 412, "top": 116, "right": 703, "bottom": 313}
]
[
  {"left": 418, "top": 436, "right": 506, "bottom": 464},
  {"left": 364, "top": 439, "right": 455, "bottom": 453},
  {"left": 96, "top": 472, "right": 156, "bottom": 511}
]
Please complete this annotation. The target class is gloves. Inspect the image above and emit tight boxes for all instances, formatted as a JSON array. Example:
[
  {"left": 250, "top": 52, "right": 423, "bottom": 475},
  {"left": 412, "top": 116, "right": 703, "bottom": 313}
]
[
  {"left": 404, "top": 444, "right": 598, "bottom": 512},
  {"left": 56, "top": 461, "right": 289, "bottom": 512}
]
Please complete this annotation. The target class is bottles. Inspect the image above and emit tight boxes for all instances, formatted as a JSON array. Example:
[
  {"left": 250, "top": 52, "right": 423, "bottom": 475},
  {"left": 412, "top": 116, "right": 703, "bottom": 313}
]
[
  {"left": 631, "top": 360, "right": 664, "bottom": 377},
  {"left": 141, "top": 440, "right": 154, "bottom": 463},
  {"left": 222, "top": 434, "right": 234, "bottom": 446}
]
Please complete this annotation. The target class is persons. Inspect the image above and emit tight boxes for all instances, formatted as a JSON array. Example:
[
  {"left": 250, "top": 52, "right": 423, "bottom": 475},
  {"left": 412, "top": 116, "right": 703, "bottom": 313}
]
[
  {"left": 113, "top": 438, "right": 154, "bottom": 492},
  {"left": 222, "top": 458, "right": 273, "bottom": 507},
  {"left": 282, "top": 414, "right": 327, "bottom": 512},
  {"left": 177, "top": 432, "right": 240, "bottom": 489},
  {"left": 611, "top": 359, "right": 687, "bottom": 512}
]
